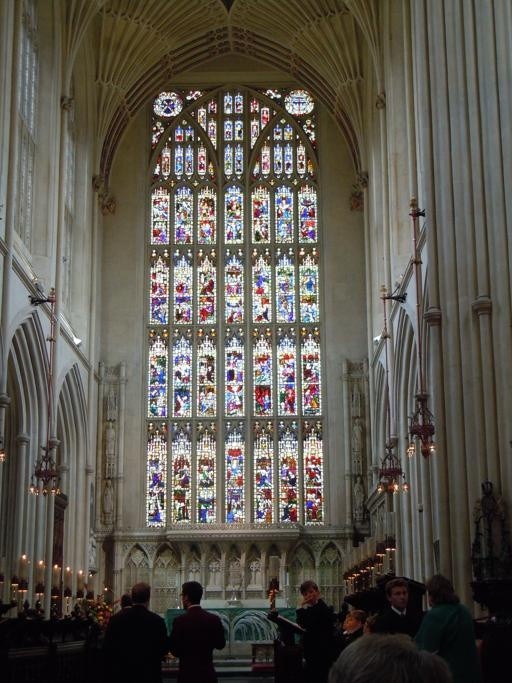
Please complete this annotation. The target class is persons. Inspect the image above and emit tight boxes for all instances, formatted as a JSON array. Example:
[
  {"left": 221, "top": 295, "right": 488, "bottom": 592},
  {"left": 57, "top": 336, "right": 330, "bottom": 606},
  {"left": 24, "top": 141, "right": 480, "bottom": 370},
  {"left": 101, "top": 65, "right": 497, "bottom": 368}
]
[
  {"left": 109, "top": 594, "right": 133, "bottom": 623},
  {"left": 296, "top": 575, "right": 481, "bottom": 683},
  {"left": 169, "top": 582, "right": 225, "bottom": 683},
  {"left": 103, "top": 421, "right": 116, "bottom": 456},
  {"left": 102, "top": 479, "right": 113, "bottom": 524},
  {"left": 101, "top": 584, "right": 170, "bottom": 683},
  {"left": 353, "top": 477, "right": 365, "bottom": 512},
  {"left": 351, "top": 381, "right": 365, "bottom": 419},
  {"left": 351, "top": 418, "right": 365, "bottom": 451},
  {"left": 106, "top": 389, "right": 117, "bottom": 421}
]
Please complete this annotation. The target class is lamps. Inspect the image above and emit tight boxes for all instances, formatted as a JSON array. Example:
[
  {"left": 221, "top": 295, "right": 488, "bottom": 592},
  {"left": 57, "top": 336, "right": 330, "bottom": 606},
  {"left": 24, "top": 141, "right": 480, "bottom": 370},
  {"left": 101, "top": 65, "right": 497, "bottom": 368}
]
[
  {"left": 30, "top": 446, "right": 62, "bottom": 496},
  {"left": 376, "top": 392, "right": 438, "bottom": 495}
]
